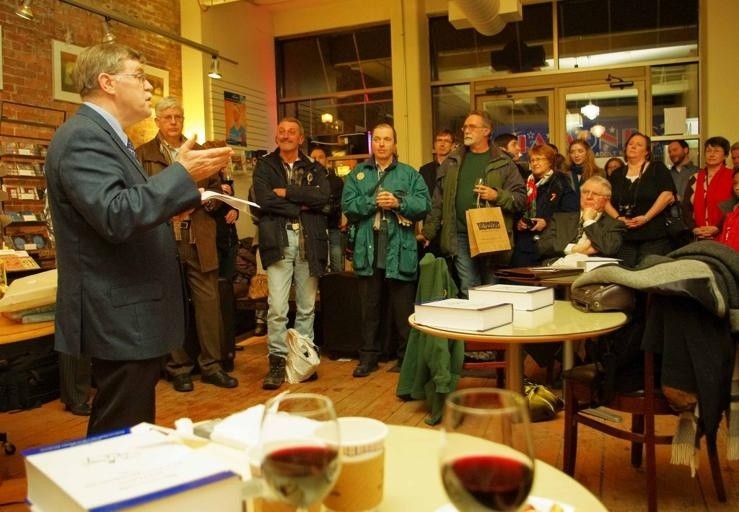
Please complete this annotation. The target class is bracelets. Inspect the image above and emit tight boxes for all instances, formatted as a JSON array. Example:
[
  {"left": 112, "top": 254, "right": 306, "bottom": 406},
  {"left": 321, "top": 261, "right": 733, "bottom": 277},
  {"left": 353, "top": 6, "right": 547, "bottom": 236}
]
[{"left": 616, "top": 216, "right": 621, "bottom": 220}]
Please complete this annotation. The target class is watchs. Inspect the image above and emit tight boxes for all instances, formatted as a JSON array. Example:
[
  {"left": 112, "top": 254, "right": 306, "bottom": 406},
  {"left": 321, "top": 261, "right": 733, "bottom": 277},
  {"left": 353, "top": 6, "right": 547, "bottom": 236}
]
[{"left": 644, "top": 215, "right": 649, "bottom": 223}]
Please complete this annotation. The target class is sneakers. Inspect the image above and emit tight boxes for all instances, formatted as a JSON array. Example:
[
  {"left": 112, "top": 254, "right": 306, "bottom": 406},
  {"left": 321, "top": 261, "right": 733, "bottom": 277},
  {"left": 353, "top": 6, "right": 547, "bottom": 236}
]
[{"left": 262, "top": 353, "right": 286, "bottom": 386}]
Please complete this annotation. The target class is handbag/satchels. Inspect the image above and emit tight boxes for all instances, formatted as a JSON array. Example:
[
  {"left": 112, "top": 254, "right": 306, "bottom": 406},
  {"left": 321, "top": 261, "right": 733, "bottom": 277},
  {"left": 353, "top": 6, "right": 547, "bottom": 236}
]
[
  {"left": 573, "top": 284, "right": 637, "bottom": 311},
  {"left": 466, "top": 197, "right": 513, "bottom": 258},
  {"left": 656, "top": 190, "right": 688, "bottom": 240}
]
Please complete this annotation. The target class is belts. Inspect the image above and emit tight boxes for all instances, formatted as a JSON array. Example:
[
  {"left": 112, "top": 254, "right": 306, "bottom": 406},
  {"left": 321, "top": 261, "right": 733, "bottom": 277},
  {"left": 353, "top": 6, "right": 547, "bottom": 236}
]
[
  {"left": 180, "top": 220, "right": 191, "bottom": 229},
  {"left": 284, "top": 224, "right": 307, "bottom": 231}
]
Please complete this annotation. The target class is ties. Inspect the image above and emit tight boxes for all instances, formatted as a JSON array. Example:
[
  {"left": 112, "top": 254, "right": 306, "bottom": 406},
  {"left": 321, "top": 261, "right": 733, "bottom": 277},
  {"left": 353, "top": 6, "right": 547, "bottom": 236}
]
[
  {"left": 128, "top": 140, "right": 138, "bottom": 161},
  {"left": 573, "top": 214, "right": 585, "bottom": 241}
]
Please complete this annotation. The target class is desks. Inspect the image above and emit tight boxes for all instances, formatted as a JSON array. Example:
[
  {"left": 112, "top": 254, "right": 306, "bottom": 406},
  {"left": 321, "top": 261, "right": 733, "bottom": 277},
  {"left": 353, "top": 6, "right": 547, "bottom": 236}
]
[
  {"left": 504, "top": 273, "right": 622, "bottom": 424},
  {"left": 409, "top": 298, "right": 628, "bottom": 393}
]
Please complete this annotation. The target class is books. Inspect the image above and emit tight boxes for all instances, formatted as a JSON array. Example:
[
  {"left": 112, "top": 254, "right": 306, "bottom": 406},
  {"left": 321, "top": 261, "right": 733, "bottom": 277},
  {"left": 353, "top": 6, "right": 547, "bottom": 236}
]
[
  {"left": 547, "top": 257, "right": 624, "bottom": 273},
  {"left": 492, "top": 266, "right": 584, "bottom": 281},
  {"left": 21, "top": 424, "right": 247, "bottom": 512},
  {"left": 413, "top": 297, "right": 513, "bottom": 332},
  {"left": 468, "top": 283, "right": 554, "bottom": 311}
]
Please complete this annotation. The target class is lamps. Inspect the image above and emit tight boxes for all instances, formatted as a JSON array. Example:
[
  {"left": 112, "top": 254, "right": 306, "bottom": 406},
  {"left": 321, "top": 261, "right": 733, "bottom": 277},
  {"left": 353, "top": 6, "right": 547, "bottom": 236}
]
[
  {"left": 580, "top": 99, "right": 598, "bottom": 119},
  {"left": 101, "top": 21, "right": 116, "bottom": 46},
  {"left": 321, "top": 113, "right": 333, "bottom": 127},
  {"left": 208, "top": 57, "right": 221, "bottom": 79},
  {"left": 591, "top": 124, "right": 605, "bottom": 138},
  {"left": 15, "top": 0, "right": 32, "bottom": 20}
]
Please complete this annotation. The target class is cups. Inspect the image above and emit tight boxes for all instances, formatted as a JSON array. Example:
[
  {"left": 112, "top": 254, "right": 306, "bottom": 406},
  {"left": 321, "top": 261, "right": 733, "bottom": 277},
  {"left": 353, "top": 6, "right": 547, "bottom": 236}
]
[{"left": 322, "top": 414, "right": 387, "bottom": 512}]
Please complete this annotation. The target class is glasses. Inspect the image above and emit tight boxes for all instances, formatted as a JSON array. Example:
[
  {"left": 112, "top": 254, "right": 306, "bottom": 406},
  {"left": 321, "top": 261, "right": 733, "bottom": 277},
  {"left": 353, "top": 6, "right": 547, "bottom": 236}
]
[
  {"left": 111, "top": 71, "right": 147, "bottom": 81},
  {"left": 462, "top": 126, "right": 488, "bottom": 132},
  {"left": 159, "top": 115, "right": 184, "bottom": 122},
  {"left": 530, "top": 156, "right": 550, "bottom": 163}
]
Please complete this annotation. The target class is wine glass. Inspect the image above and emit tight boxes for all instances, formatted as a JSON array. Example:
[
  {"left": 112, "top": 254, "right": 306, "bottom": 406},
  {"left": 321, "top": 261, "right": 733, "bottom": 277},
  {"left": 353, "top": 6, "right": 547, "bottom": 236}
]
[
  {"left": 441, "top": 389, "right": 534, "bottom": 512},
  {"left": 259, "top": 392, "right": 342, "bottom": 511}
]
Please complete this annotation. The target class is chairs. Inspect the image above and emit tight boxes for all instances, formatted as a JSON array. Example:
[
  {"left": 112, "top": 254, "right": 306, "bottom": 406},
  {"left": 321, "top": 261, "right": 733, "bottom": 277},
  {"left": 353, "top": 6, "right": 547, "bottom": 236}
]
[
  {"left": 418, "top": 253, "right": 506, "bottom": 388},
  {"left": 561, "top": 292, "right": 726, "bottom": 512}
]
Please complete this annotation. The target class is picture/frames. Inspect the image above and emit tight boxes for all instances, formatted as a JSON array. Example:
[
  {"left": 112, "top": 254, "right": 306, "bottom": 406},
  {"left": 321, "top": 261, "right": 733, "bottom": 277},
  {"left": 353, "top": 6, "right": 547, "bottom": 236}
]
[
  {"left": 142, "top": 67, "right": 169, "bottom": 119},
  {"left": 51, "top": 40, "right": 88, "bottom": 105}
]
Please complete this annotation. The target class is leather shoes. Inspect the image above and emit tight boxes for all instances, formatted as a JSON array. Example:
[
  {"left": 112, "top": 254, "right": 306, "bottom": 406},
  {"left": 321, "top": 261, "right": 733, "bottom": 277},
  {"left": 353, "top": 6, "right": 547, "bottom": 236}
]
[
  {"left": 353, "top": 361, "right": 379, "bottom": 377},
  {"left": 173, "top": 371, "right": 193, "bottom": 391},
  {"left": 67, "top": 401, "right": 92, "bottom": 415},
  {"left": 201, "top": 371, "right": 238, "bottom": 390}
]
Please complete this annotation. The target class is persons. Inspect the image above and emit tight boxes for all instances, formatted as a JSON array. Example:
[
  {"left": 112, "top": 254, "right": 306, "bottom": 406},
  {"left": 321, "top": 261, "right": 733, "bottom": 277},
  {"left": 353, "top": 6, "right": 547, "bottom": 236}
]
[
  {"left": 310, "top": 146, "right": 348, "bottom": 360},
  {"left": 418, "top": 128, "right": 457, "bottom": 259},
  {"left": 340, "top": 121, "right": 432, "bottom": 377},
  {"left": 252, "top": 117, "right": 330, "bottom": 389},
  {"left": 201, "top": 141, "right": 239, "bottom": 373},
  {"left": 604, "top": 157, "right": 625, "bottom": 177},
  {"left": 602, "top": 133, "right": 679, "bottom": 268},
  {"left": 730, "top": 142, "right": 739, "bottom": 173},
  {"left": 663, "top": 140, "right": 702, "bottom": 255},
  {"left": 133, "top": 97, "right": 239, "bottom": 391},
  {"left": 712, "top": 168, "right": 739, "bottom": 255},
  {"left": 415, "top": 109, "right": 527, "bottom": 301},
  {"left": 44, "top": 44, "right": 234, "bottom": 438},
  {"left": 561, "top": 139, "right": 607, "bottom": 185},
  {"left": 512, "top": 145, "right": 580, "bottom": 268},
  {"left": 493, "top": 133, "right": 527, "bottom": 185},
  {"left": 681, "top": 137, "right": 735, "bottom": 243},
  {"left": 522, "top": 175, "right": 626, "bottom": 389}
]
